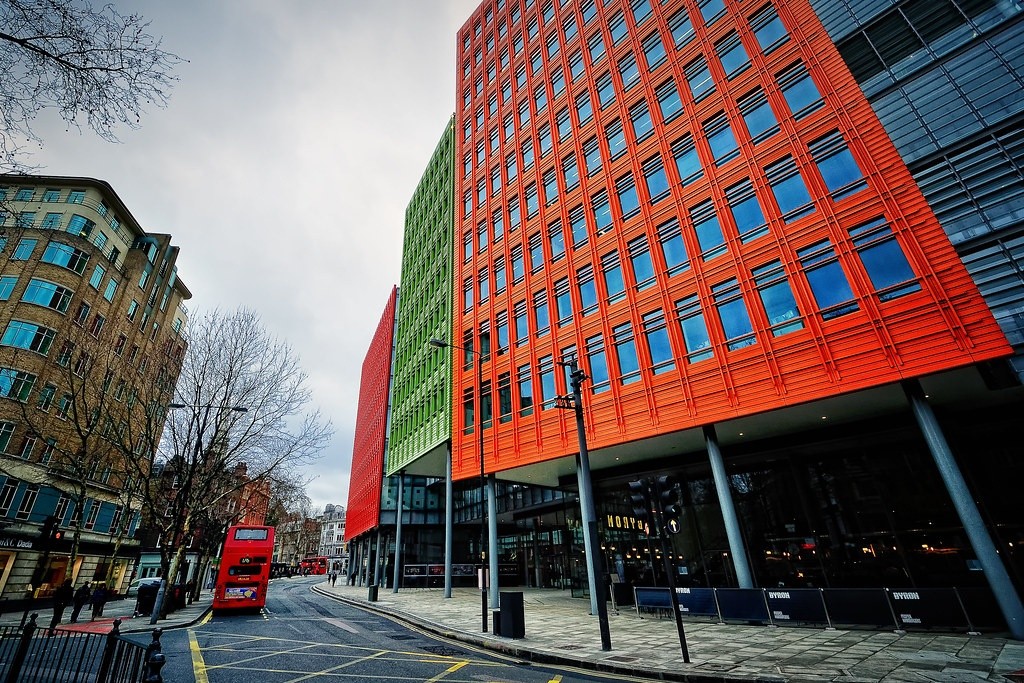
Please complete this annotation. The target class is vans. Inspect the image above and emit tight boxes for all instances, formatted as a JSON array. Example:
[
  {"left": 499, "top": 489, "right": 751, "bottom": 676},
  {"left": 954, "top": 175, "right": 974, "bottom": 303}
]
[{"left": 126, "top": 577, "right": 163, "bottom": 596}]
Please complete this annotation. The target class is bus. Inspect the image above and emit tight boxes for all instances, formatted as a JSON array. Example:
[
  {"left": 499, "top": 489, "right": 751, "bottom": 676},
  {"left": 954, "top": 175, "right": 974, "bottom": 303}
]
[
  {"left": 300, "top": 557, "right": 326, "bottom": 575},
  {"left": 212, "top": 524, "right": 275, "bottom": 617}
]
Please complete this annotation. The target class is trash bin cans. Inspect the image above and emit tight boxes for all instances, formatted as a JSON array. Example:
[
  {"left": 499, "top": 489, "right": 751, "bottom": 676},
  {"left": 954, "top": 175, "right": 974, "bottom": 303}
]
[
  {"left": 368, "top": 585, "right": 378, "bottom": 602},
  {"left": 500, "top": 592, "right": 525, "bottom": 638},
  {"left": 133, "top": 582, "right": 181, "bottom": 618}
]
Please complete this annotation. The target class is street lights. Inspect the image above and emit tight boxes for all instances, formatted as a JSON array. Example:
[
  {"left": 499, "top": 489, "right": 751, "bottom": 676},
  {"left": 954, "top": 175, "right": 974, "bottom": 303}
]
[
  {"left": 427, "top": 338, "right": 489, "bottom": 635},
  {"left": 150, "top": 402, "right": 249, "bottom": 626}
]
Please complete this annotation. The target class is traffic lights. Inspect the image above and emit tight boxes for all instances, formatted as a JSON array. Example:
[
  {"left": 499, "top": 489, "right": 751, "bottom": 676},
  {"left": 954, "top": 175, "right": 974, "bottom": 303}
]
[
  {"left": 34, "top": 516, "right": 58, "bottom": 550},
  {"left": 50, "top": 531, "right": 66, "bottom": 550}
]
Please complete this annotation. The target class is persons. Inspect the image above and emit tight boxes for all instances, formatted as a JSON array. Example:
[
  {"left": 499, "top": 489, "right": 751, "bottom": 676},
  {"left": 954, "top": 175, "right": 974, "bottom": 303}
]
[
  {"left": 48, "top": 579, "right": 109, "bottom": 636},
  {"left": 271, "top": 567, "right": 308, "bottom": 579},
  {"left": 326, "top": 563, "right": 395, "bottom": 590}
]
